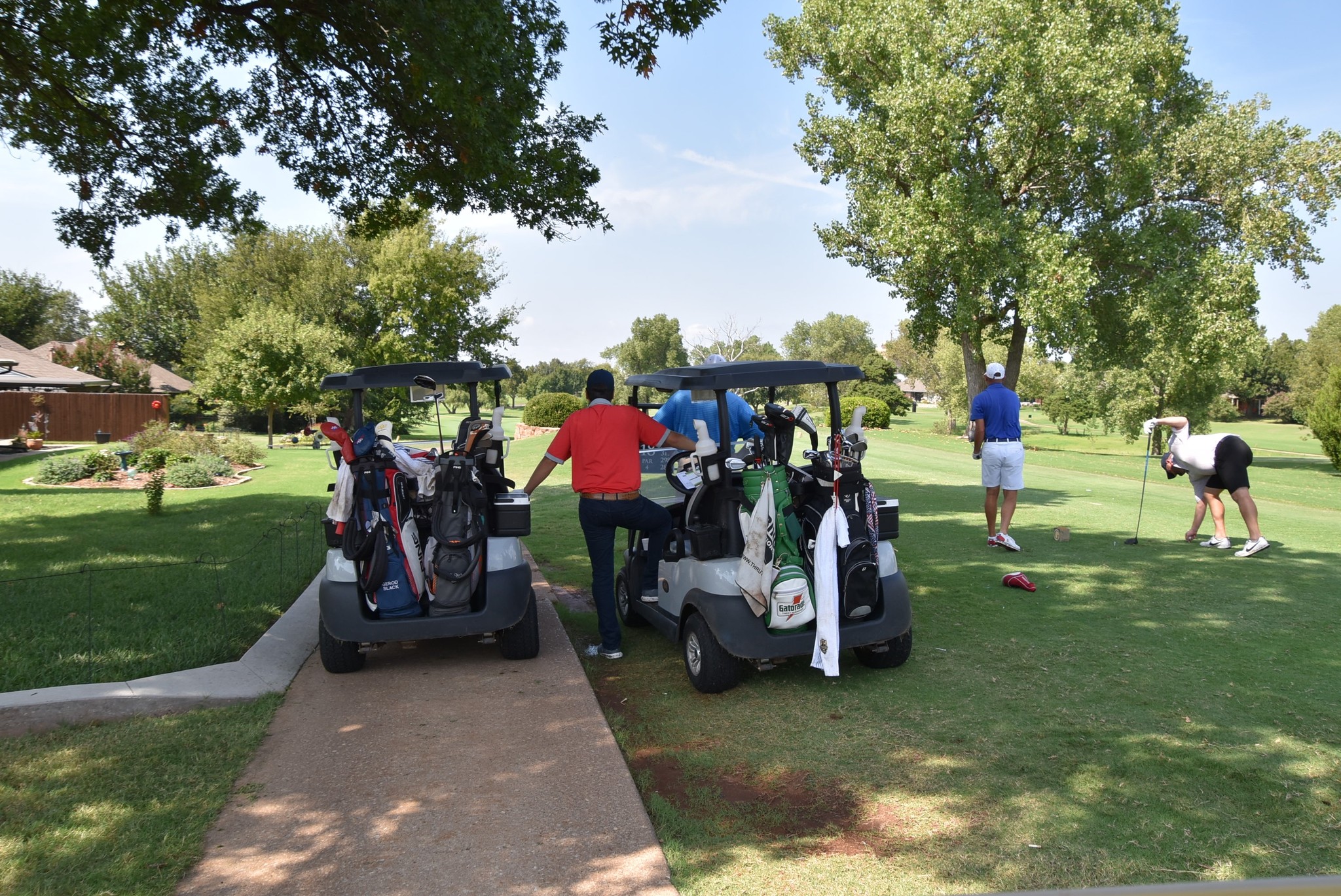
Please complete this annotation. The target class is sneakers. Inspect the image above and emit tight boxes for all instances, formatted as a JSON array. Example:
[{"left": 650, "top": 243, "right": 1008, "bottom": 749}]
[
  {"left": 995, "top": 533, "right": 1021, "bottom": 551},
  {"left": 1234, "top": 537, "right": 1270, "bottom": 557},
  {"left": 597, "top": 644, "right": 623, "bottom": 659},
  {"left": 1199, "top": 535, "right": 1230, "bottom": 548},
  {"left": 987, "top": 533, "right": 998, "bottom": 547},
  {"left": 641, "top": 589, "right": 658, "bottom": 602}
]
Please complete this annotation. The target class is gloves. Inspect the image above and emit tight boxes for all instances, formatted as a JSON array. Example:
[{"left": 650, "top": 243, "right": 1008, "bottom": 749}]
[
  {"left": 1143, "top": 417, "right": 1157, "bottom": 434},
  {"left": 512, "top": 488, "right": 525, "bottom": 498},
  {"left": 972, "top": 451, "right": 982, "bottom": 459}
]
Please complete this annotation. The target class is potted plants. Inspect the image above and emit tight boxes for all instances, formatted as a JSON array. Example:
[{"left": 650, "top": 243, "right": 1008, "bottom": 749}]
[
  {"left": 11, "top": 436, "right": 23, "bottom": 447},
  {"left": 25, "top": 432, "right": 44, "bottom": 450}
]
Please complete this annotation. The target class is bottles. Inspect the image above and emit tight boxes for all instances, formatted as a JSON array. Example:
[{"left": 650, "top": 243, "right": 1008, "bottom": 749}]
[
  {"left": 689, "top": 419, "right": 720, "bottom": 480},
  {"left": 485, "top": 406, "right": 510, "bottom": 464}
]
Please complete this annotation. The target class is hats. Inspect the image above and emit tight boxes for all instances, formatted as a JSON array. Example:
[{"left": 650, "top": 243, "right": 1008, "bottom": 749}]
[
  {"left": 984, "top": 363, "right": 1006, "bottom": 380},
  {"left": 587, "top": 369, "right": 614, "bottom": 391},
  {"left": 1161, "top": 450, "right": 1176, "bottom": 479}
]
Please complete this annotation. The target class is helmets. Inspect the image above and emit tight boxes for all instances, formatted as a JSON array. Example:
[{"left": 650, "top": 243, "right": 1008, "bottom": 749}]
[{"left": 704, "top": 353, "right": 727, "bottom": 363}]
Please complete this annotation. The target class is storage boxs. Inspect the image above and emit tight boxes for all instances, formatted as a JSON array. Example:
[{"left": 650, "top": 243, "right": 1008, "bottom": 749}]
[
  {"left": 876, "top": 496, "right": 899, "bottom": 540},
  {"left": 491, "top": 492, "right": 531, "bottom": 537}
]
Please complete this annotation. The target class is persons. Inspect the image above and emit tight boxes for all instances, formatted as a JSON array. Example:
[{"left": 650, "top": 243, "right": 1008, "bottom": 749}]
[
  {"left": 970, "top": 363, "right": 1025, "bottom": 552},
  {"left": 1142, "top": 416, "right": 1269, "bottom": 556},
  {"left": 524, "top": 355, "right": 766, "bottom": 659}
]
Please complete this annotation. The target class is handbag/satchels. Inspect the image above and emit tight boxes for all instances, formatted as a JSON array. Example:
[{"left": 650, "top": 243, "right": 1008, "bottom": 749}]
[
  {"left": 429, "top": 535, "right": 482, "bottom": 607},
  {"left": 763, "top": 564, "right": 816, "bottom": 632},
  {"left": 365, "top": 536, "right": 422, "bottom": 619}
]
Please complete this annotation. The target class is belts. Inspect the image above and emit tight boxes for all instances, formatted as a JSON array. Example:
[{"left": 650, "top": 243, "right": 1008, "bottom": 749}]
[
  {"left": 984, "top": 438, "right": 1021, "bottom": 442},
  {"left": 581, "top": 490, "right": 639, "bottom": 501}
]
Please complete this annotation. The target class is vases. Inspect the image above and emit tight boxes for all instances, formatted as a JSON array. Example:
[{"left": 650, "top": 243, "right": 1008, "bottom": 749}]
[{"left": 93, "top": 432, "right": 111, "bottom": 444}]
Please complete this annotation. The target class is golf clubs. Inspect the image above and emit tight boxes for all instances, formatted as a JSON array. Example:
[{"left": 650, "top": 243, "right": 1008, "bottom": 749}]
[
  {"left": 454, "top": 432, "right": 495, "bottom": 457},
  {"left": 724, "top": 436, "right": 770, "bottom": 470},
  {"left": 413, "top": 374, "right": 447, "bottom": 457},
  {"left": 1123, "top": 427, "right": 1153, "bottom": 546},
  {"left": 802, "top": 433, "right": 868, "bottom": 468}
]
